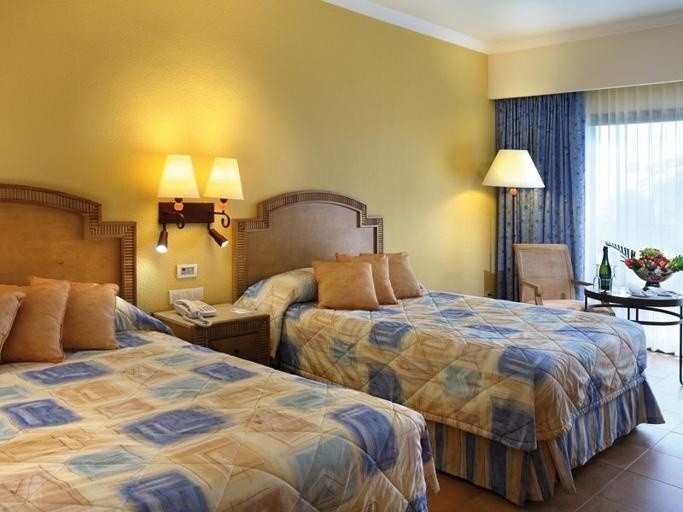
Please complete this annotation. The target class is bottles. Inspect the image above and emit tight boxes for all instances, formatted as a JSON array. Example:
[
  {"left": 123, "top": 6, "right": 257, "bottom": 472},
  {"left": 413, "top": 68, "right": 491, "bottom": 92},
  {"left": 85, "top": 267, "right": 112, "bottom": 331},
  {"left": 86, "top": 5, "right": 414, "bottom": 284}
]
[{"left": 599, "top": 246, "right": 612, "bottom": 290}]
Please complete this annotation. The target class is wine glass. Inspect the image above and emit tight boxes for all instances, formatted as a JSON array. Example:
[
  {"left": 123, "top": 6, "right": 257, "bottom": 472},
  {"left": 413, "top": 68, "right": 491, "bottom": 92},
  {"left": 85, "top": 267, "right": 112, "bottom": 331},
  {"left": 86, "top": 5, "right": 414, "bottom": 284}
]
[
  {"left": 592, "top": 263, "right": 617, "bottom": 291},
  {"left": 634, "top": 267, "right": 672, "bottom": 293}
]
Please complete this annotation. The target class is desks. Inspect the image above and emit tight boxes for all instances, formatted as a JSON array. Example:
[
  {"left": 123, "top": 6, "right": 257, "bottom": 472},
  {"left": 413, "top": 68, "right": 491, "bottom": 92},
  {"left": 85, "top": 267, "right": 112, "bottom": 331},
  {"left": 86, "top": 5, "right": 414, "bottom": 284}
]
[{"left": 584, "top": 286, "right": 683, "bottom": 386}]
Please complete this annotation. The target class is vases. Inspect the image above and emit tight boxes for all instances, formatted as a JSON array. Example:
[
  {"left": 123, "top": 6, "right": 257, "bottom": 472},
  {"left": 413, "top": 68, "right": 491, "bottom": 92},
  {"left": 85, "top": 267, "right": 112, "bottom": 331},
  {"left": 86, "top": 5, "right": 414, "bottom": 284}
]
[{"left": 644, "top": 279, "right": 661, "bottom": 291}]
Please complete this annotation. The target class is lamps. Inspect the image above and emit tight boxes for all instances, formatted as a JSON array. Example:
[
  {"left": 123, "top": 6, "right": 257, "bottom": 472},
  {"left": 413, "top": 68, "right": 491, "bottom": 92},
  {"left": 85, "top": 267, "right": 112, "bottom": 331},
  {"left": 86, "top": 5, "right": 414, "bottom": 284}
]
[
  {"left": 481, "top": 148, "right": 546, "bottom": 301},
  {"left": 155, "top": 154, "right": 244, "bottom": 254}
]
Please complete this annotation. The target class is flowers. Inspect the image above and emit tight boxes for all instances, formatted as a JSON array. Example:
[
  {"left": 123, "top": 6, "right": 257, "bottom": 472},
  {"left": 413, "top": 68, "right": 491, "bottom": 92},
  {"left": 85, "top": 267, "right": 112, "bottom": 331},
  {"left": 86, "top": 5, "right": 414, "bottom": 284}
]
[{"left": 606, "top": 240, "right": 683, "bottom": 281}]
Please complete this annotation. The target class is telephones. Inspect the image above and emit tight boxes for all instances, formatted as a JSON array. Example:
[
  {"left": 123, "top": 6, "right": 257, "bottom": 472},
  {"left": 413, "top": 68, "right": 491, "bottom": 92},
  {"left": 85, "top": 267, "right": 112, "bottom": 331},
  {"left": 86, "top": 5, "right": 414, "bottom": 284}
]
[{"left": 174, "top": 299, "right": 217, "bottom": 318}]
